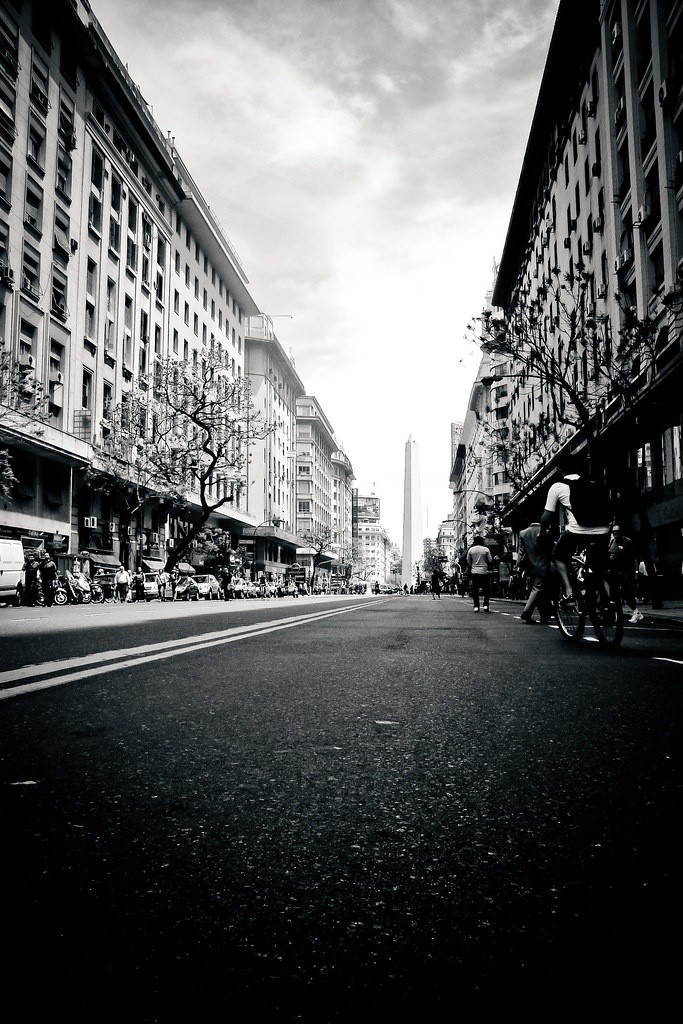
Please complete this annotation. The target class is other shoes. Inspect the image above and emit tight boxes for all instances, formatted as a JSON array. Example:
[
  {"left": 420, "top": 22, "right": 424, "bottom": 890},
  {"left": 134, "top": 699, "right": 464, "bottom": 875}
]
[
  {"left": 474, "top": 605, "right": 489, "bottom": 613},
  {"left": 521, "top": 612, "right": 535, "bottom": 624},
  {"left": 628, "top": 611, "right": 643, "bottom": 623},
  {"left": 558, "top": 594, "right": 575, "bottom": 608},
  {"left": 539, "top": 616, "right": 549, "bottom": 624}
]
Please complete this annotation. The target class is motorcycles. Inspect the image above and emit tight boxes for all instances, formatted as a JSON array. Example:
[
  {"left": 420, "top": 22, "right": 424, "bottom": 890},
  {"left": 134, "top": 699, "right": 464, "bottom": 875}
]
[{"left": 36, "top": 570, "right": 104, "bottom": 606}]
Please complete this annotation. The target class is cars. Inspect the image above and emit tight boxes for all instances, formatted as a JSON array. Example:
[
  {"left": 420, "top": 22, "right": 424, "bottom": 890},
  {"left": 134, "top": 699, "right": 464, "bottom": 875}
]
[
  {"left": 96, "top": 575, "right": 118, "bottom": 599},
  {"left": 219, "top": 578, "right": 308, "bottom": 599},
  {"left": 177, "top": 576, "right": 200, "bottom": 601},
  {"left": 131, "top": 572, "right": 173, "bottom": 601}
]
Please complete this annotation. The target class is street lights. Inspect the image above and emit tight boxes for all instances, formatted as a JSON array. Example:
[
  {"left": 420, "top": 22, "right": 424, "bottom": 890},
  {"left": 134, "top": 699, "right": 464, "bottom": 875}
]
[
  {"left": 252, "top": 519, "right": 285, "bottom": 581},
  {"left": 443, "top": 519, "right": 475, "bottom": 545},
  {"left": 453, "top": 489, "right": 495, "bottom": 543}
]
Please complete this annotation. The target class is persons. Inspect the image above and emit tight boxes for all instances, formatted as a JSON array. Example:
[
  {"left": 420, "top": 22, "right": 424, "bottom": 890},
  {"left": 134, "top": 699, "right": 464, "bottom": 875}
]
[
  {"left": 22, "top": 550, "right": 384, "bottom": 604},
  {"left": 466, "top": 537, "right": 494, "bottom": 613},
  {"left": 403, "top": 455, "right": 661, "bottom": 626}
]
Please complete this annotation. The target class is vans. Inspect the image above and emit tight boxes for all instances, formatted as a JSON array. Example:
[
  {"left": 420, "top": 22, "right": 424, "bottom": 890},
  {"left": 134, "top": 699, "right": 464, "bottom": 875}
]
[{"left": 0, "top": 538, "right": 27, "bottom": 606}]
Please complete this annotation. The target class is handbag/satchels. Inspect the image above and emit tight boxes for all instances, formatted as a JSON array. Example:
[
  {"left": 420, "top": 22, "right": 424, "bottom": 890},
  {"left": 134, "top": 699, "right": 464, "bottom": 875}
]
[{"left": 520, "top": 554, "right": 533, "bottom": 570}]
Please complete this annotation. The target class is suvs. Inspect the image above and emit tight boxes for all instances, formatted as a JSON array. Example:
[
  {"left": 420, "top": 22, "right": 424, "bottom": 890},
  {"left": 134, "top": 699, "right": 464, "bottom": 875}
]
[{"left": 191, "top": 574, "right": 221, "bottom": 600}]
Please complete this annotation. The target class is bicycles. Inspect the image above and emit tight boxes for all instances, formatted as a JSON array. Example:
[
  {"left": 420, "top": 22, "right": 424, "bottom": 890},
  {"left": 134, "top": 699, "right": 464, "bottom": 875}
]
[{"left": 535, "top": 530, "right": 624, "bottom": 647}]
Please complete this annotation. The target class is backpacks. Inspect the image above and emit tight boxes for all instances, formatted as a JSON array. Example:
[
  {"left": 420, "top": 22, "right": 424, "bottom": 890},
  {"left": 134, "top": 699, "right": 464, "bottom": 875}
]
[{"left": 557, "top": 476, "right": 612, "bottom": 529}]
